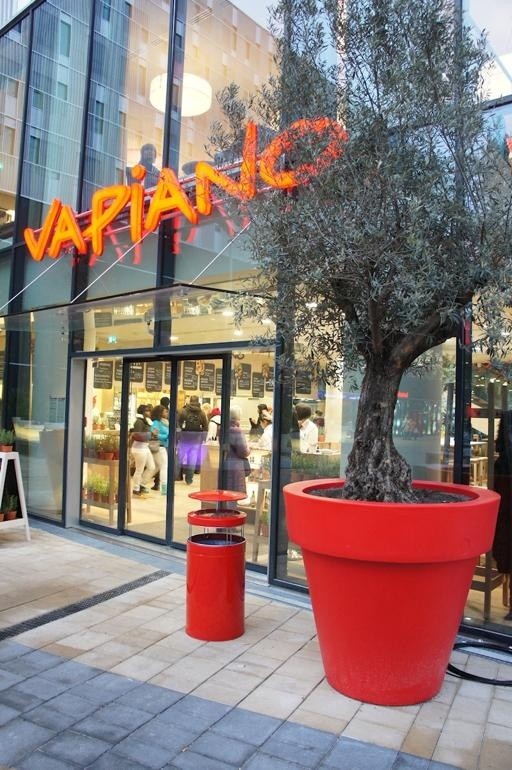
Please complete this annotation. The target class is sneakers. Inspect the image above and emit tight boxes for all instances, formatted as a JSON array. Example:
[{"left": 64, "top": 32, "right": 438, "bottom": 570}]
[
  {"left": 176, "top": 471, "right": 200, "bottom": 484},
  {"left": 133, "top": 486, "right": 148, "bottom": 495}
]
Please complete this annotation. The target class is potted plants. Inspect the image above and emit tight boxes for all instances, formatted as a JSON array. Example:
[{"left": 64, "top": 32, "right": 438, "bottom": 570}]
[
  {"left": 259, "top": 510, "right": 269, "bottom": 538},
  {"left": 204, "top": 0, "right": 512, "bottom": 707},
  {"left": 82, "top": 470, "right": 119, "bottom": 504},
  {"left": 0, "top": 488, "right": 18, "bottom": 522},
  {"left": 262, "top": 449, "right": 340, "bottom": 483},
  {"left": 0, "top": 428, "right": 17, "bottom": 452},
  {"left": 83, "top": 433, "right": 120, "bottom": 460}
]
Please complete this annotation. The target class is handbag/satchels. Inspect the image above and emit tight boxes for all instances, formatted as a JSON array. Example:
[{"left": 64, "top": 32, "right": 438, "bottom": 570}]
[
  {"left": 149, "top": 439, "right": 161, "bottom": 452},
  {"left": 244, "top": 458, "right": 251, "bottom": 477}
]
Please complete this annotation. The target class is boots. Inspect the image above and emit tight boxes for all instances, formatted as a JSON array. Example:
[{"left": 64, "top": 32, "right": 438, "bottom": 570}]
[{"left": 151, "top": 474, "right": 160, "bottom": 490}]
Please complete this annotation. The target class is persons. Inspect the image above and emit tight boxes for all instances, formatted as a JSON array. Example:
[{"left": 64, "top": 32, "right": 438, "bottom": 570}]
[
  {"left": 402, "top": 413, "right": 424, "bottom": 440},
  {"left": 131, "top": 395, "right": 222, "bottom": 495},
  {"left": 215, "top": 405, "right": 251, "bottom": 533},
  {"left": 471, "top": 433, "right": 482, "bottom": 457},
  {"left": 250, "top": 397, "right": 324, "bottom": 453},
  {"left": 132, "top": 144, "right": 161, "bottom": 188}
]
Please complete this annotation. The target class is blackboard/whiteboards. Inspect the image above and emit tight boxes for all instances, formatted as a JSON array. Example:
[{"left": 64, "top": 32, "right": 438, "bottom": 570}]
[{"left": 94, "top": 359, "right": 311, "bottom": 399}]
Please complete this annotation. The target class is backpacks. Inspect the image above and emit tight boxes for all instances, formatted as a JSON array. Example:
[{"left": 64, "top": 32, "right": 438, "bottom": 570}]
[{"left": 185, "top": 404, "right": 202, "bottom": 431}]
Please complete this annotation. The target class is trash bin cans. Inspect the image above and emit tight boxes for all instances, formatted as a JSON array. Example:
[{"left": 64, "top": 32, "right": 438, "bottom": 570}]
[{"left": 186, "top": 488, "right": 249, "bottom": 641}]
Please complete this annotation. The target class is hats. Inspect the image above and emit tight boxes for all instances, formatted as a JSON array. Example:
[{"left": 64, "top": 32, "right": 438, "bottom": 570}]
[
  {"left": 212, "top": 408, "right": 220, "bottom": 416},
  {"left": 231, "top": 409, "right": 240, "bottom": 421}
]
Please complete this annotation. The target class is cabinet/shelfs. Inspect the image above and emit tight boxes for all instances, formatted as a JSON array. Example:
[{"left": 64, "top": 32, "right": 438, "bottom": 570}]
[
  {"left": 0, "top": 452, "right": 31, "bottom": 541},
  {"left": 250, "top": 479, "right": 302, "bottom": 562},
  {"left": 80, "top": 430, "right": 132, "bottom": 525}
]
